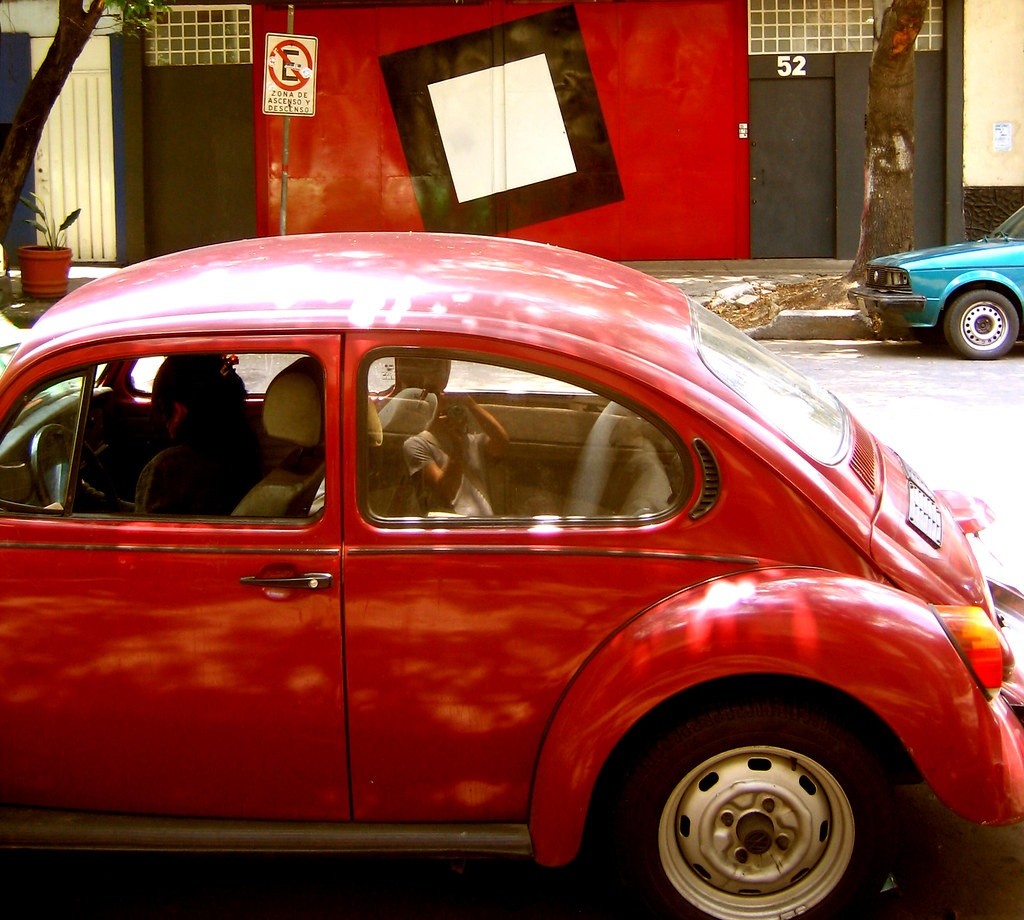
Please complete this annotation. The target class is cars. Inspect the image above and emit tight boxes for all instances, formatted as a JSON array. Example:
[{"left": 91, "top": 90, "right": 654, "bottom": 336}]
[
  {"left": 0, "top": 231, "right": 1024, "bottom": 920},
  {"left": 846, "top": 203, "right": 1023, "bottom": 361}
]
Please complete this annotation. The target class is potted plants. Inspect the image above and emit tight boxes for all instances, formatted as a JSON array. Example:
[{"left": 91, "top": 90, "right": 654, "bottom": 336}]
[{"left": 18, "top": 190, "right": 83, "bottom": 300}]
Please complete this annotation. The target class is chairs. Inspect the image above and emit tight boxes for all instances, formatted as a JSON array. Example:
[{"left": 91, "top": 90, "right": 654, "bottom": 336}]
[
  {"left": 368, "top": 358, "right": 452, "bottom": 517},
  {"left": 221, "top": 356, "right": 327, "bottom": 519}
]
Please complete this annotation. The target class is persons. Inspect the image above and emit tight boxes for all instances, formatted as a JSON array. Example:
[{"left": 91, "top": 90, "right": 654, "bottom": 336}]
[
  {"left": 62, "top": 349, "right": 264, "bottom": 522},
  {"left": 400, "top": 380, "right": 513, "bottom": 518}
]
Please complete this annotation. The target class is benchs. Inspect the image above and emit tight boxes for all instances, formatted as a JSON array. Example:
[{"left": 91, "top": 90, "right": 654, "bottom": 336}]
[{"left": 601, "top": 443, "right": 673, "bottom": 519}]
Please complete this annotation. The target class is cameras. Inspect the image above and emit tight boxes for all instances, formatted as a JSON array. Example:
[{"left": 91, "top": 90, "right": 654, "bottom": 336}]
[{"left": 446, "top": 402, "right": 470, "bottom": 419}]
